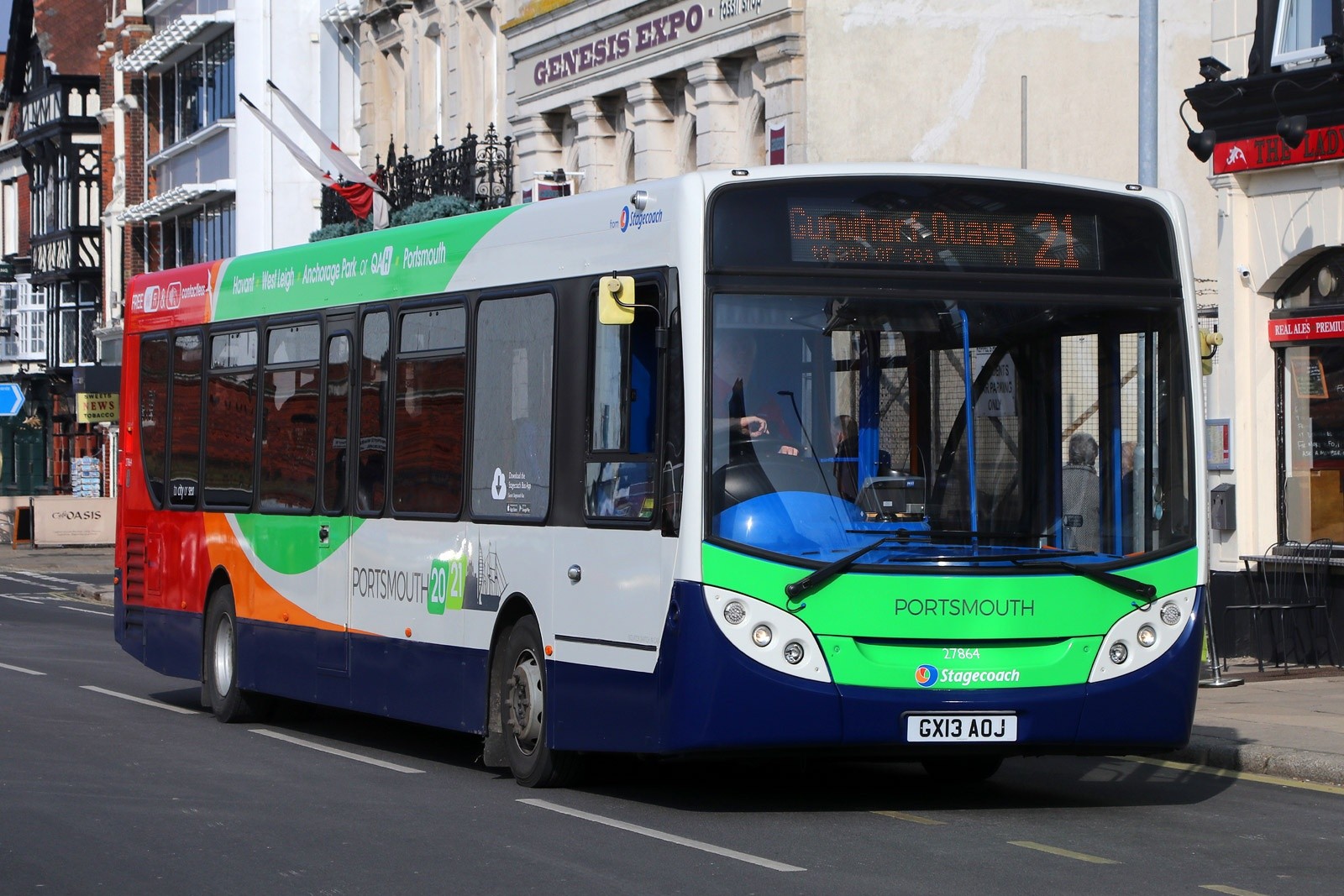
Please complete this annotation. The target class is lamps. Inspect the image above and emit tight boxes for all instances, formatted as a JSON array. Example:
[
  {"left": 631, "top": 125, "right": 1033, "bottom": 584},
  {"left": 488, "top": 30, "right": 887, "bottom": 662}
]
[
  {"left": 1271, "top": 73, "right": 1344, "bottom": 150},
  {"left": 1179, "top": 86, "right": 1248, "bottom": 163},
  {"left": 1197, "top": 56, "right": 1232, "bottom": 85},
  {"left": 1321, "top": 34, "right": 1344, "bottom": 64}
]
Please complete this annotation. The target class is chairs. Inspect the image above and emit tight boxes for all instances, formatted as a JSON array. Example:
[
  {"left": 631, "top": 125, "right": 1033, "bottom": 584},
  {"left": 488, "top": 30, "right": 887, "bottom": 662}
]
[
  {"left": 708, "top": 456, "right": 820, "bottom": 520},
  {"left": 1221, "top": 539, "right": 1302, "bottom": 672},
  {"left": 1259, "top": 538, "right": 1344, "bottom": 674}
]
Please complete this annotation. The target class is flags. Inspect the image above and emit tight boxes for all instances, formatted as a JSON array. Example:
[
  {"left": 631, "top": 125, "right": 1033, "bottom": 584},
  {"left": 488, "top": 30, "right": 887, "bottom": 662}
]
[{"left": 242, "top": 84, "right": 390, "bottom": 231}]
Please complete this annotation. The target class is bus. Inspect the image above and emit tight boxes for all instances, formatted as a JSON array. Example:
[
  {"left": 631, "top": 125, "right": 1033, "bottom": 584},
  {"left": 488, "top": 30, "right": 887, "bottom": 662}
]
[{"left": 112, "top": 159, "right": 1225, "bottom": 789}]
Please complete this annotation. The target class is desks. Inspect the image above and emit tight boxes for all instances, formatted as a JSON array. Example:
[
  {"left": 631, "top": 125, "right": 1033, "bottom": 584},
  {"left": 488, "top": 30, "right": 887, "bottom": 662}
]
[{"left": 1237, "top": 554, "right": 1343, "bottom": 654}]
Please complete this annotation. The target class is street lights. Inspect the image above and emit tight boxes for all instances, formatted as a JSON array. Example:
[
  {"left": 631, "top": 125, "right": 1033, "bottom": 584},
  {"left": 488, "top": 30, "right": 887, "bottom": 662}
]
[{"left": 774, "top": 388, "right": 852, "bottom": 546}]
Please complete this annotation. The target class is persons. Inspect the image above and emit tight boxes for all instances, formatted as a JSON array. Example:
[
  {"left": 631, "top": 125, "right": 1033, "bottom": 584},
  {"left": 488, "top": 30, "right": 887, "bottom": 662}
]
[
  {"left": 1061, "top": 432, "right": 1100, "bottom": 552},
  {"left": 831, "top": 414, "right": 859, "bottom": 500},
  {"left": 648, "top": 330, "right": 801, "bottom": 468},
  {"left": 1120, "top": 441, "right": 1137, "bottom": 555}
]
